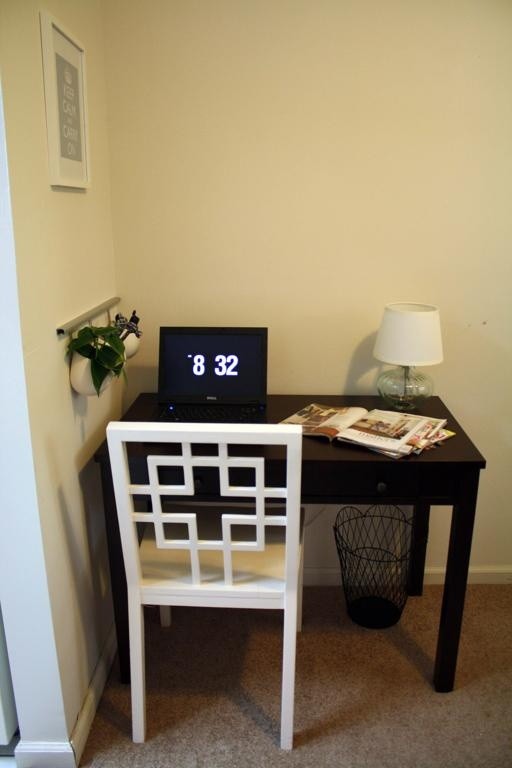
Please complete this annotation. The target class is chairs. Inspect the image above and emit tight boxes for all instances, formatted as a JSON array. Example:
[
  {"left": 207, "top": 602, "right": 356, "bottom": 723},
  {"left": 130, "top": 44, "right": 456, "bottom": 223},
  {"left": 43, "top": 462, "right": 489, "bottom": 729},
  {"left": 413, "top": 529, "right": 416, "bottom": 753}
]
[{"left": 106, "top": 422, "right": 305, "bottom": 751}]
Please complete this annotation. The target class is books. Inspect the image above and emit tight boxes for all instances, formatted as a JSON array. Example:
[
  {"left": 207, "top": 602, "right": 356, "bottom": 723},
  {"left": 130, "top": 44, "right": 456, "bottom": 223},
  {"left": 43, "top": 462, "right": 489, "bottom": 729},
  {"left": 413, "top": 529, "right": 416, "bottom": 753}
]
[{"left": 280, "top": 403, "right": 456, "bottom": 459}]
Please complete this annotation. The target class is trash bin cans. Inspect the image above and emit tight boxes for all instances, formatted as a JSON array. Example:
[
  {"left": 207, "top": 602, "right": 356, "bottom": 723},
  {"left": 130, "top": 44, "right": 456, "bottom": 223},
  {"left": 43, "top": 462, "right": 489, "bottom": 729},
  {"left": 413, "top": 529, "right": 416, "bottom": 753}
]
[{"left": 332, "top": 503, "right": 414, "bottom": 630}]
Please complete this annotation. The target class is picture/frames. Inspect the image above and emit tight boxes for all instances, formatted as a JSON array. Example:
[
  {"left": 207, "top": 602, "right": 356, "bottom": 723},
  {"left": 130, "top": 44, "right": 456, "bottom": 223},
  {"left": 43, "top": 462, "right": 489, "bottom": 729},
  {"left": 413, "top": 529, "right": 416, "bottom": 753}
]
[{"left": 40, "top": 9, "right": 92, "bottom": 189}]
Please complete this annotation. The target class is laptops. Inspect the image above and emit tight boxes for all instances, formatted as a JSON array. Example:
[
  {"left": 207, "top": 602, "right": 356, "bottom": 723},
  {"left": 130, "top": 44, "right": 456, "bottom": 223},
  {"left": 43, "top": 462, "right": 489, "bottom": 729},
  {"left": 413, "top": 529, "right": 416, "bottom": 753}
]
[{"left": 152, "top": 326, "right": 268, "bottom": 423}]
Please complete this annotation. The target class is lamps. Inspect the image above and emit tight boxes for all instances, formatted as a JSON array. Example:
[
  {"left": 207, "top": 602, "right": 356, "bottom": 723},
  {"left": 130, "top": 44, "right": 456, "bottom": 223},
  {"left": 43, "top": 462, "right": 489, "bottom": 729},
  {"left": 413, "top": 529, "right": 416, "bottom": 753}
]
[{"left": 370, "top": 303, "right": 444, "bottom": 412}]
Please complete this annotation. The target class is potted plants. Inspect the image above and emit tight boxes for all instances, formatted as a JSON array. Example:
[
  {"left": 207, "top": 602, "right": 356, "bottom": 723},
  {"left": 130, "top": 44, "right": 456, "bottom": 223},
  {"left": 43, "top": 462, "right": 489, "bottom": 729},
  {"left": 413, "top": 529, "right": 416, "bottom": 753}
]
[{"left": 65, "top": 326, "right": 130, "bottom": 398}]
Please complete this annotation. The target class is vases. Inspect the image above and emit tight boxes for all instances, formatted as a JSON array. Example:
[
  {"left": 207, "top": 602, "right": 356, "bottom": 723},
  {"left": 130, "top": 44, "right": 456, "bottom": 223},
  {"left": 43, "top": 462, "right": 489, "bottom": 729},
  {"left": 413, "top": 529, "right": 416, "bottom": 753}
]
[{"left": 120, "top": 327, "right": 143, "bottom": 357}]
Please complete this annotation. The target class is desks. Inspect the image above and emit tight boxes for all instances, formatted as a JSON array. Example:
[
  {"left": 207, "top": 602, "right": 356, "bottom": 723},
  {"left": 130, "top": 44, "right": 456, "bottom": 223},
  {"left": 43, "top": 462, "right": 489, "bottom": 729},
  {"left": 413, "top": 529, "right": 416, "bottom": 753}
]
[{"left": 92, "top": 395, "right": 486, "bottom": 692}]
